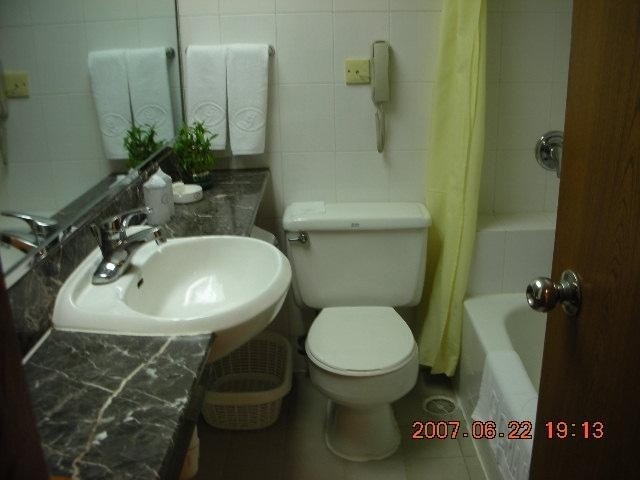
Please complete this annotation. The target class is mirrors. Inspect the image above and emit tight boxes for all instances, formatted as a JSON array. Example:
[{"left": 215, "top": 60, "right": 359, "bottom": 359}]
[{"left": 0, "top": 1, "right": 185, "bottom": 294}]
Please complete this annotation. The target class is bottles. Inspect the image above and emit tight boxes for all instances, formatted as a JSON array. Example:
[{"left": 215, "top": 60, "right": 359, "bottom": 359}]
[{"left": 143, "top": 169, "right": 175, "bottom": 225}]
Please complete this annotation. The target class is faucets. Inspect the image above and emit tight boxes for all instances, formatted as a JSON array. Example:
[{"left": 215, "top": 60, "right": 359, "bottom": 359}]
[
  {"left": 89, "top": 207, "right": 167, "bottom": 285},
  {"left": 0, "top": 210, "right": 59, "bottom": 257}
]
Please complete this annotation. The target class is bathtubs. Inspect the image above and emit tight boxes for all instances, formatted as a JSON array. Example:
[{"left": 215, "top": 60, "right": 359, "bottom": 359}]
[{"left": 452, "top": 291, "right": 547, "bottom": 477}]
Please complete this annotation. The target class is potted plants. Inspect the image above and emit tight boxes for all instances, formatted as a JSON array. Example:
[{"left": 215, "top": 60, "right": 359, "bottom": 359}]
[{"left": 171, "top": 118, "right": 218, "bottom": 190}]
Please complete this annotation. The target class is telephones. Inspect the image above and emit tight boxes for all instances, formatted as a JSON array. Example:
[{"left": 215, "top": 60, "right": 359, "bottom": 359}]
[{"left": 371, "top": 40, "right": 390, "bottom": 105}]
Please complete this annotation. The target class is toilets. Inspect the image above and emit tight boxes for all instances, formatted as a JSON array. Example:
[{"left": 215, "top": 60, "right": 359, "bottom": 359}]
[{"left": 282, "top": 200, "right": 432, "bottom": 461}]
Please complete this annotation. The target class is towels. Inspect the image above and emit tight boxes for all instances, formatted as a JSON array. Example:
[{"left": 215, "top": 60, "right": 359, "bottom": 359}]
[
  {"left": 182, "top": 44, "right": 269, "bottom": 156},
  {"left": 87, "top": 47, "right": 176, "bottom": 161},
  {"left": 470, "top": 347, "right": 539, "bottom": 480}
]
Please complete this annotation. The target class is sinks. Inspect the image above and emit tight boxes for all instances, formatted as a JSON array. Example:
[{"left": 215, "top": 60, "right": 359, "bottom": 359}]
[{"left": 51, "top": 222, "right": 292, "bottom": 337}]
